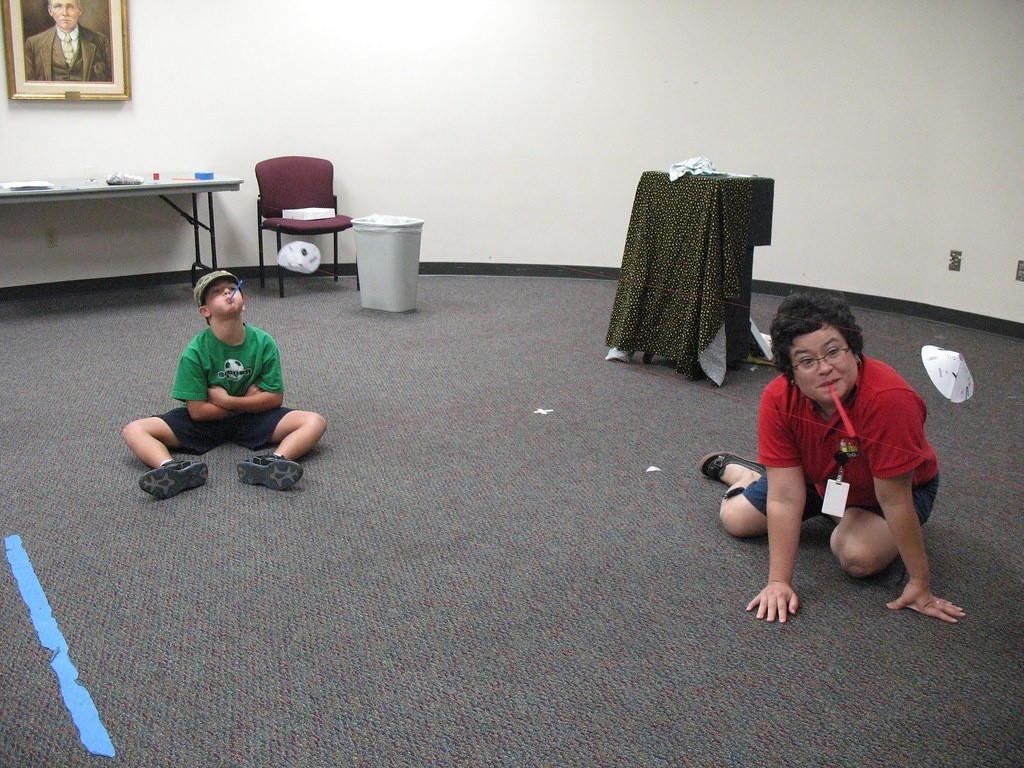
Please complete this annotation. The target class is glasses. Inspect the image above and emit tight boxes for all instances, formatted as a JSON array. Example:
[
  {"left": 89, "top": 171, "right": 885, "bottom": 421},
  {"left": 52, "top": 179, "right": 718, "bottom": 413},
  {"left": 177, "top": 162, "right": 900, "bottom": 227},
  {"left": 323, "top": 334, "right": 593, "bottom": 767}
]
[{"left": 791, "top": 344, "right": 850, "bottom": 373}]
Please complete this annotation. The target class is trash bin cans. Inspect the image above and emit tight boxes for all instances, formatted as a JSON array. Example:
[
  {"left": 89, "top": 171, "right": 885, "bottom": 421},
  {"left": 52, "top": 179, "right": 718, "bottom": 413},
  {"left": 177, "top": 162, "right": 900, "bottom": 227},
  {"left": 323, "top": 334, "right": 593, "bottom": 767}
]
[{"left": 351, "top": 215, "right": 424, "bottom": 312}]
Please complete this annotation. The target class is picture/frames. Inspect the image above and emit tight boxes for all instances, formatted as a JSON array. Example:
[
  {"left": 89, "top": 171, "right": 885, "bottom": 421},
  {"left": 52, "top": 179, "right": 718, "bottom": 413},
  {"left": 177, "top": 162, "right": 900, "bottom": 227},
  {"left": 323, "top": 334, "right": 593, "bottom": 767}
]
[{"left": 2, "top": 0, "right": 132, "bottom": 102}]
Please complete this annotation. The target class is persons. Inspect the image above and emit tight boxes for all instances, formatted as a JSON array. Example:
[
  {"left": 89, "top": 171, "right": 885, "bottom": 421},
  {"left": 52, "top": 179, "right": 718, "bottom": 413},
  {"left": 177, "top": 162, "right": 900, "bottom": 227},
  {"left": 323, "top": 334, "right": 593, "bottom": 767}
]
[
  {"left": 700, "top": 290, "right": 967, "bottom": 623},
  {"left": 122, "top": 270, "right": 328, "bottom": 500}
]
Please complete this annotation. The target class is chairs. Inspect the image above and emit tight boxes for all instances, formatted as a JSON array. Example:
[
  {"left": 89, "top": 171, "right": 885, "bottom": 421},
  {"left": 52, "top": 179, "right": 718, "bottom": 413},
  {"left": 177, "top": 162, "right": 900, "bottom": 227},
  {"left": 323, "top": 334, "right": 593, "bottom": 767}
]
[{"left": 254, "top": 156, "right": 360, "bottom": 298}]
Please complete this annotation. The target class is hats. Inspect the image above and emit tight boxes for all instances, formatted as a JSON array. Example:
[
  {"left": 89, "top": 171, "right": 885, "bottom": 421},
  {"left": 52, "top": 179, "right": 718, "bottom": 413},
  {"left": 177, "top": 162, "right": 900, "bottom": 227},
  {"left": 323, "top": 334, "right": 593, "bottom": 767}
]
[{"left": 193, "top": 270, "right": 243, "bottom": 306}]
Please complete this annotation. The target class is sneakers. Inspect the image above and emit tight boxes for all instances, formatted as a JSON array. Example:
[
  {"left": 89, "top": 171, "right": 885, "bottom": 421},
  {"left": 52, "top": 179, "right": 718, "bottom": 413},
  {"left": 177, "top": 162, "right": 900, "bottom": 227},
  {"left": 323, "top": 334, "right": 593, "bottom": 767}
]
[
  {"left": 138, "top": 459, "right": 209, "bottom": 499},
  {"left": 236, "top": 453, "right": 303, "bottom": 491}
]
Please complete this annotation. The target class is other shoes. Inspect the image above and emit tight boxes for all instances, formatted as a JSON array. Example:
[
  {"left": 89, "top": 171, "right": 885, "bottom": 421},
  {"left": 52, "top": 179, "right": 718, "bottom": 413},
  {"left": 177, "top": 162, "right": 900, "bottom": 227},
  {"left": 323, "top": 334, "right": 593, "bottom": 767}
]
[{"left": 699, "top": 451, "right": 765, "bottom": 487}]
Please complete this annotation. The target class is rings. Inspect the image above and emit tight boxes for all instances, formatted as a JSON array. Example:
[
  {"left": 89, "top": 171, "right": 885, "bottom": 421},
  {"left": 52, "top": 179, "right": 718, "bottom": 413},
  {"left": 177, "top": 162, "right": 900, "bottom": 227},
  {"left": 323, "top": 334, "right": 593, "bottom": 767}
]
[{"left": 937, "top": 601, "right": 940, "bottom": 604}]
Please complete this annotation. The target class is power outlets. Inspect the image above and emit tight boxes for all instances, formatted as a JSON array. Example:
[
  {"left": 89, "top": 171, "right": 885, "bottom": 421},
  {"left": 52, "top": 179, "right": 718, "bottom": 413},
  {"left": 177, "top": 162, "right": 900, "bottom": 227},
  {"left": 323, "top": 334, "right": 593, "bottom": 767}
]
[
  {"left": 1016, "top": 260, "right": 1024, "bottom": 281},
  {"left": 47, "top": 228, "right": 59, "bottom": 248},
  {"left": 948, "top": 250, "right": 962, "bottom": 271}
]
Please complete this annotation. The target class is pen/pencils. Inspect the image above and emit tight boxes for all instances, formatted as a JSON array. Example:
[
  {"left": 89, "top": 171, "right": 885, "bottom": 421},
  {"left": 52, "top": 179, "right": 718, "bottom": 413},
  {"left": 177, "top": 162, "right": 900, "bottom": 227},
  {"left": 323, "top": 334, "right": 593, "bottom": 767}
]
[{"left": 172, "top": 178, "right": 199, "bottom": 180}]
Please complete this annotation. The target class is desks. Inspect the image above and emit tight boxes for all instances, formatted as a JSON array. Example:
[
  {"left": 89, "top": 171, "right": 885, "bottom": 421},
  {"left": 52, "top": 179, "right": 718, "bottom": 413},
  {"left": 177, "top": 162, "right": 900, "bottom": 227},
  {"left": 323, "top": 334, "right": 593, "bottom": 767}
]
[
  {"left": 0, "top": 173, "right": 244, "bottom": 290},
  {"left": 600, "top": 172, "right": 776, "bottom": 386}
]
